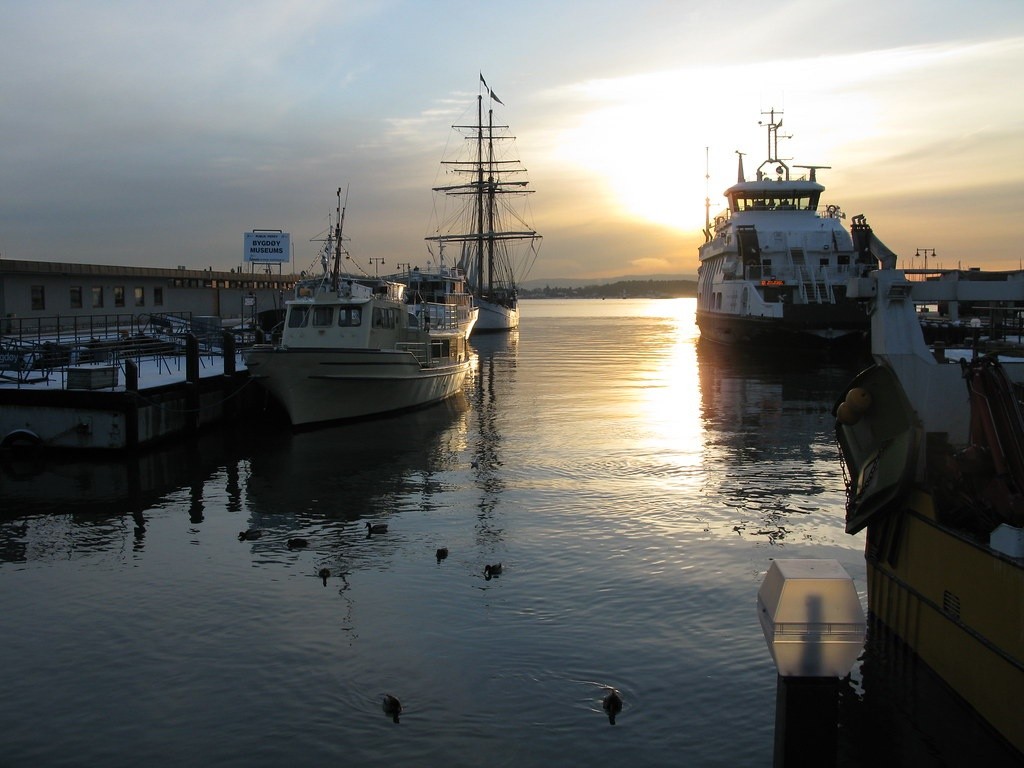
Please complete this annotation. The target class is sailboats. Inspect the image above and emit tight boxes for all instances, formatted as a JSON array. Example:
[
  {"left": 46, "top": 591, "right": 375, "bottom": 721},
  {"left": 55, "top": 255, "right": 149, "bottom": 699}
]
[{"left": 410, "top": 71, "right": 545, "bottom": 325}]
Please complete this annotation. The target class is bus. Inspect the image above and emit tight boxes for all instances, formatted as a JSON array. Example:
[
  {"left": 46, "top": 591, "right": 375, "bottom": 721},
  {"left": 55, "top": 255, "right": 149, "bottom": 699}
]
[
  {"left": 980, "top": 317, "right": 992, "bottom": 326},
  {"left": 1002, "top": 319, "right": 1020, "bottom": 329}
]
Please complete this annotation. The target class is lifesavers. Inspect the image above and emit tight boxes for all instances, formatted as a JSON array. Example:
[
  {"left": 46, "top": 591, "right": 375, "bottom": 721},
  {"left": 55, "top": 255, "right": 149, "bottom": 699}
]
[{"left": 746, "top": 259, "right": 757, "bottom": 268}]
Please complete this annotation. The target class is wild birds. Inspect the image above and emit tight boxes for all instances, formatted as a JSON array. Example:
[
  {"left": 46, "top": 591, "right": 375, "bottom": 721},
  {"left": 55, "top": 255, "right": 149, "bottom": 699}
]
[
  {"left": 286, "top": 538, "right": 308, "bottom": 549},
  {"left": 318, "top": 566, "right": 331, "bottom": 578},
  {"left": 382, "top": 693, "right": 404, "bottom": 718},
  {"left": 237, "top": 528, "right": 263, "bottom": 541},
  {"left": 603, "top": 688, "right": 623, "bottom": 716},
  {"left": 483, "top": 562, "right": 503, "bottom": 578},
  {"left": 435, "top": 546, "right": 450, "bottom": 561},
  {"left": 364, "top": 520, "right": 389, "bottom": 536}
]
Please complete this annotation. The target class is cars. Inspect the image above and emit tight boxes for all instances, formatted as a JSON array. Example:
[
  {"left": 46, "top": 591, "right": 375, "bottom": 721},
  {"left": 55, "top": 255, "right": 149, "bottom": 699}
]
[{"left": 920, "top": 317, "right": 971, "bottom": 328}]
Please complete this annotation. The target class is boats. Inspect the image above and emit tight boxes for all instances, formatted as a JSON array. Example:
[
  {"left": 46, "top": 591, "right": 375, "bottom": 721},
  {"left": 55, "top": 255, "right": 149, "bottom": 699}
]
[
  {"left": 831, "top": 316, "right": 1024, "bottom": 756},
  {"left": 693, "top": 107, "right": 899, "bottom": 336},
  {"left": 240, "top": 187, "right": 482, "bottom": 429}
]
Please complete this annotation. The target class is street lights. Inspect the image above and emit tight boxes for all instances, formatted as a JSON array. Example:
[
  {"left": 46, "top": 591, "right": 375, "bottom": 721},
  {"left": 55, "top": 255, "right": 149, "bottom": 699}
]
[
  {"left": 397, "top": 262, "right": 410, "bottom": 281},
  {"left": 367, "top": 257, "right": 385, "bottom": 278},
  {"left": 915, "top": 246, "right": 937, "bottom": 281}
]
